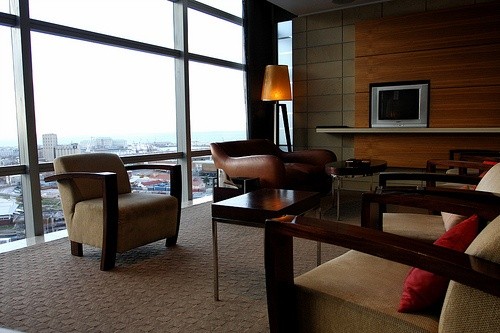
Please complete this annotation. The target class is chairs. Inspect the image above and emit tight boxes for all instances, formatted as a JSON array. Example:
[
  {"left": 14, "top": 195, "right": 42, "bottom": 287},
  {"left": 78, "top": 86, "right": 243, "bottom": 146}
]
[
  {"left": 211, "top": 139, "right": 337, "bottom": 217},
  {"left": 42, "top": 153, "right": 183, "bottom": 272},
  {"left": 425, "top": 147, "right": 500, "bottom": 188},
  {"left": 263, "top": 188, "right": 500, "bottom": 333},
  {"left": 380, "top": 171, "right": 500, "bottom": 237}
]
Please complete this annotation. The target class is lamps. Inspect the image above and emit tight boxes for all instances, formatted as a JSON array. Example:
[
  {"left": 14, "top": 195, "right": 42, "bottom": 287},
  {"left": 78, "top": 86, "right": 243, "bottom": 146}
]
[{"left": 259, "top": 64, "right": 292, "bottom": 146}]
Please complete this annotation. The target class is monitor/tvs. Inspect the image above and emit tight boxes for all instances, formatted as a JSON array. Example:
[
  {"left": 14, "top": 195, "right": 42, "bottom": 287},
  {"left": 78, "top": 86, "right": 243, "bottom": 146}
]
[{"left": 370, "top": 83, "right": 429, "bottom": 129}]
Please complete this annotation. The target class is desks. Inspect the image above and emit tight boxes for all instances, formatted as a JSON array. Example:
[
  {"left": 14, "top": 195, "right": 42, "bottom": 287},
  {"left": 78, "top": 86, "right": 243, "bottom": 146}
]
[
  {"left": 324, "top": 158, "right": 387, "bottom": 222},
  {"left": 210, "top": 187, "right": 326, "bottom": 302},
  {"left": 213, "top": 182, "right": 269, "bottom": 202}
]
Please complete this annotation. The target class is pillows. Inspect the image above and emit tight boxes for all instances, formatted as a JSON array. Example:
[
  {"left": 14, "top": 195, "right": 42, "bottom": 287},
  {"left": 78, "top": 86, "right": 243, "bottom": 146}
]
[
  {"left": 481, "top": 161, "right": 498, "bottom": 166},
  {"left": 397, "top": 213, "right": 480, "bottom": 316},
  {"left": 440, "top": 211, "right": 465, "bottom": 232}
]
